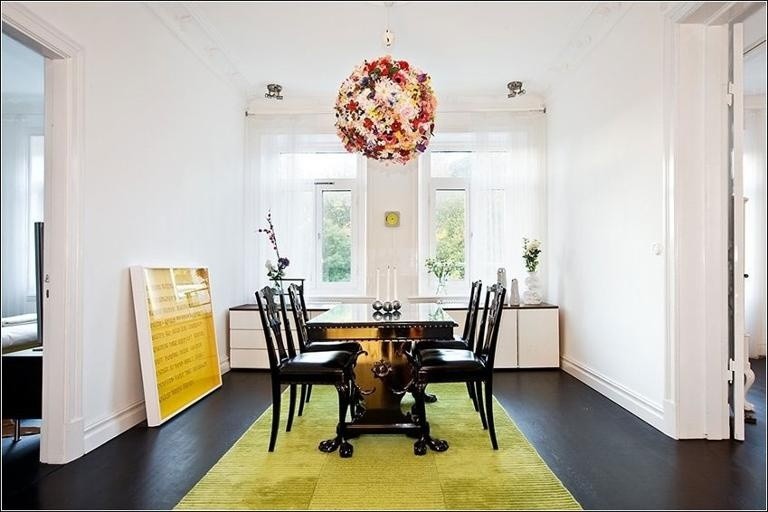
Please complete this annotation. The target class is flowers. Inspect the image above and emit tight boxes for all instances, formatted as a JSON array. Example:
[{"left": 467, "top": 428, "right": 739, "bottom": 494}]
[
  {"left": 520, "top": 233, "right": 544, "bottom": 271},
  {"left": 258, "top": 208, "right": 288, "bottom": 287}
]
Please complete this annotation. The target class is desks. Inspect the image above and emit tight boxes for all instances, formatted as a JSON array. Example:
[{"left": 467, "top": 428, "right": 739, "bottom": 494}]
[{"left": 305, "top": 303, "right": 458, "bottom": 458}]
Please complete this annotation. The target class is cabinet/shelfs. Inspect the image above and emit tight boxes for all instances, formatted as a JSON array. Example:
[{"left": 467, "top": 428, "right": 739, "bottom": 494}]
[
  {"left": 405, "top": 297, "right": 562, "bottom": 370},
  {"left": 2, "top": 344, "right": 43, "bottom": 443},
  {"left": 229, "top": 303, "right": 376, "bottom": 372}
]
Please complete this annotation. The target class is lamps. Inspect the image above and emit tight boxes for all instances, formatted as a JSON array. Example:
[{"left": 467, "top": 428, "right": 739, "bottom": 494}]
[
  {"left": 265, "top": 84, "right": 283, "bottom": 100},
  {"left": 333, "top": 1, "right": 437, "bottom": 165},
  {"left": 506, "top": 80, "right": 526, "bottom": 98}
]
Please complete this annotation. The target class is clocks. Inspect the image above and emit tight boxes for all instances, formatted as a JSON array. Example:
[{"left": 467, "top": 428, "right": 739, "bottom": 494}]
[{"left": 383, "top": 211, "right": 400, "bottom": 229}]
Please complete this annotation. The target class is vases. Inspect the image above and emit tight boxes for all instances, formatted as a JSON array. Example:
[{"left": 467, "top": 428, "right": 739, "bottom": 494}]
[{"left": 522, "top": 269, "right": 543, "bottom": 304}]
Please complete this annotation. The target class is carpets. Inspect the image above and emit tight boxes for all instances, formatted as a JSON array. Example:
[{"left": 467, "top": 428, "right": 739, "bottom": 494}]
[{"left": 174, "top": 383, "right": 584, "bottom": 511}]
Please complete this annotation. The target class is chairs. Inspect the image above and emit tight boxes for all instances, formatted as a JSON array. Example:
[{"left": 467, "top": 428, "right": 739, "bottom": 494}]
[
  {"left": 412, "top": 281, "right": 506, "bottom": 456},
  {"left": 287, "top": 283, "right": 361, "bottom": 420},
  {"left": 255, "top": 286, "right": 355, "bottom": 457},
  {"left": 410, "top": 279, "right": 482, "bottom": 416}
]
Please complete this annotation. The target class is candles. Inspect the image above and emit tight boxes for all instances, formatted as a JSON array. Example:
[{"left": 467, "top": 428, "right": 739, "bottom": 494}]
[
  {"left": 385, "top": 264, "right": 390, "bottom": 301},
  {"left": 375, "top": 267, "right": 380, "bottom": 301},
  {"left": 392, "top": 265, "right": 397, "bottom": 300}
]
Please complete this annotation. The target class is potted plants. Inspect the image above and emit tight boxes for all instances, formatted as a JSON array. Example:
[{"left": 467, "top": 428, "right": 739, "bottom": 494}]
[{"left": 423, "top": 253, "right": 464, "bottom": 296}]
